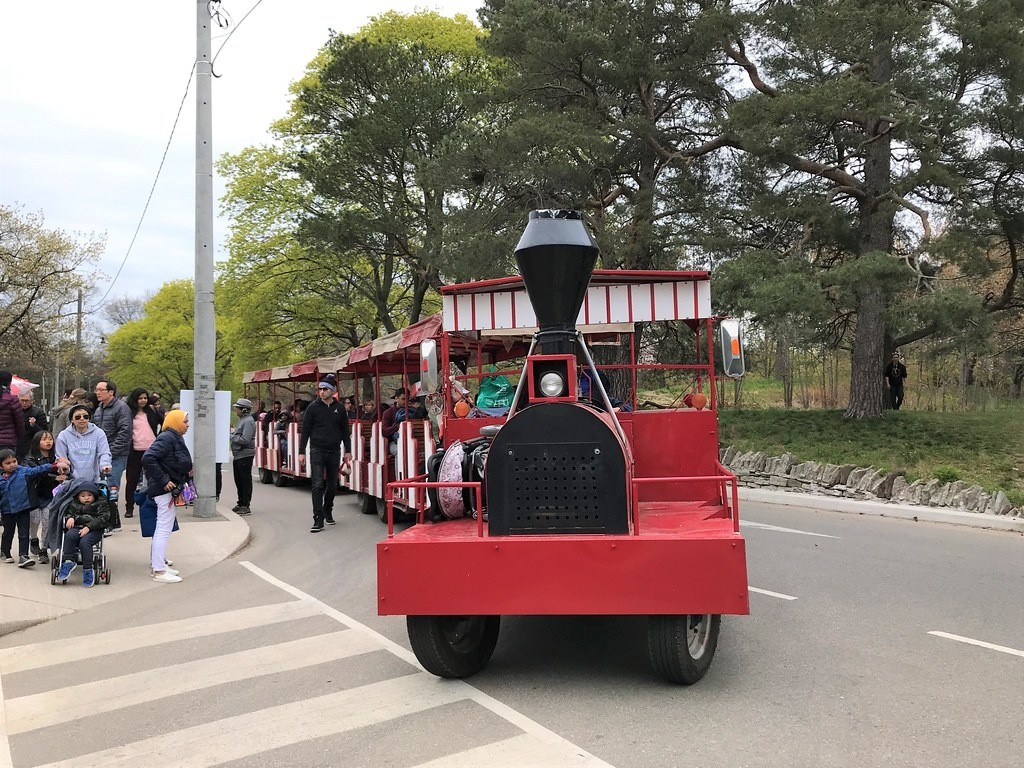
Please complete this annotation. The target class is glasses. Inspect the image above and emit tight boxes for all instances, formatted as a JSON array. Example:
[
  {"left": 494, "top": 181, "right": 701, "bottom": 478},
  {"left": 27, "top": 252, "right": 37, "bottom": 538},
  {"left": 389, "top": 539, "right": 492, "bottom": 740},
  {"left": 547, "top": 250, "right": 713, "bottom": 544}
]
[
  {"left": 72, "top": 415, "right": 90, "bottom": 420},
  {"left": 318, "top": 387, "right": 330, "bottom": 391},
  {"left": 94, "top": 388, "right": 107, "bottom": 393}
]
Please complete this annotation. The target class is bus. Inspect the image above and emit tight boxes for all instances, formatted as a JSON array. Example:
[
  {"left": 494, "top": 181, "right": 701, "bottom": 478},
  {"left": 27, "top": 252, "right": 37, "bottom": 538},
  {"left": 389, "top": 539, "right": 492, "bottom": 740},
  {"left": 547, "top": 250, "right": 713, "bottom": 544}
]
[{"left": 241, "top": 207, "right": 750, "bottom": 679}]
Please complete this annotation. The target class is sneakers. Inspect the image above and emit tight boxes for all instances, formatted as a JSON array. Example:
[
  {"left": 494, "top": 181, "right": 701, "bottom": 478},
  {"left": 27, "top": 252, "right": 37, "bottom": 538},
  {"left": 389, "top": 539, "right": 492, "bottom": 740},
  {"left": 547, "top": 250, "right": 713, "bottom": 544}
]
[
  {"left": 29, "top": 539, "right": 40, "bottom": 555},
  {"left": 324, "top": 513, "right": 335, "bottom": 525},
  {"left": 310, "top": 519, "right": 324, "bottom": 532},
  {"left": 17, "top": 556, "right": 36, "bottom": 569},
  {"left": 231, "top": 505, "right": 252, "bottom": 515},
  {"left": 38, "top": 548, "right": 48, "bottom": 564},
  {"left": 0, "top": 550, "right": 14, "bottom": 562},
  {"left": 82, "top": 569, "right": 95, "bottom": 587},
  {"left": 57, "top": 561, "right": 77, "bottom": 580}
]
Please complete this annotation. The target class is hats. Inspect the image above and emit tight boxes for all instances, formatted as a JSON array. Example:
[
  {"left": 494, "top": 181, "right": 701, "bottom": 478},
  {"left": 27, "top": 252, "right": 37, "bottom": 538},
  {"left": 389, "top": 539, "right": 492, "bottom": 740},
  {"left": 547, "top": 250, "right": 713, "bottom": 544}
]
[
  {"left": 68, "top": 404, "right": 92, "bottom": 422},
  {"left": 232, "top": 398, "right": 254, "bottom": 408},
  {"left": 390, "top": 387, "right": 410, "bottom": 399},
  {"left": 462, "top": 387, "right": 469, "bottom": 394},
  {"left": 319, "top": 373, "right": 337, "bottom": 393}
]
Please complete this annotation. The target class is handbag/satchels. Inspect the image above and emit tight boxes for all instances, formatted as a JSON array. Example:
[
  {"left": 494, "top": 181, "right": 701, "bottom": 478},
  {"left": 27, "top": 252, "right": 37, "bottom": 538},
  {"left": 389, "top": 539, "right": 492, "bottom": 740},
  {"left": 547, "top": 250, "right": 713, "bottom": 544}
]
[
  {"left": 174, "top": 479, "right": 198, "bottom": 507},
  {"left": 138, "top": 495, "right": 179, "bottom": 537}
]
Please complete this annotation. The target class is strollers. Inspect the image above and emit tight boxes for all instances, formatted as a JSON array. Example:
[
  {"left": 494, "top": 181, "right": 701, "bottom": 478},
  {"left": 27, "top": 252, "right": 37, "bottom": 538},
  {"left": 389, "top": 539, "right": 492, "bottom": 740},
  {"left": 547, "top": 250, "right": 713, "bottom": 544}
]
[{"left": 47, "top": 467, "right": 111, "bottom": 586}]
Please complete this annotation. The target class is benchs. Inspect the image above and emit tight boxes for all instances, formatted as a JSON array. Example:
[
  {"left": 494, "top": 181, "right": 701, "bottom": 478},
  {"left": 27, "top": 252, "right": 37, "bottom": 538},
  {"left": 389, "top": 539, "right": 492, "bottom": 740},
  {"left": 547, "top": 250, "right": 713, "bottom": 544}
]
[{"left": 254, "top": 421, "right": 436, "bottom": 507}]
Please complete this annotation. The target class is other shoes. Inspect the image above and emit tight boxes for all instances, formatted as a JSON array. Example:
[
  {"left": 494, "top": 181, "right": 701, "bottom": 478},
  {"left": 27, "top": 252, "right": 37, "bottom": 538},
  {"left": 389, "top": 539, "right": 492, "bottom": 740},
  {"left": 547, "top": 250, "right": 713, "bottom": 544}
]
[
  {"left": 152, "top": 567, "right": 179, "bottom": 575},
  {"left": 150, "top": 560, "right": 173, "bottom": 566},
  {"left": 152, "top": 572, "right": 182, "bottom": 583},
  {"left": 125, "top": 506, "right": 134, "bottom": 517}
]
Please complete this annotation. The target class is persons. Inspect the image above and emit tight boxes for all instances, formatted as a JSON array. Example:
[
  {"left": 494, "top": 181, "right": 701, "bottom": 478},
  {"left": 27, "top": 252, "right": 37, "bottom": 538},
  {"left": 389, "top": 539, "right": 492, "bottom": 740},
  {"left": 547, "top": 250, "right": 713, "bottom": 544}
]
[
  {"left": 228, "top": 398, "right": 256, "bottom": 515},
  {"left": 578, "top": 371, "right": 632, "bottom": 413},
  {"left": 1, "top": 380, "right": 222, "bottom": 587},
  {"left": 273, "top": 387, "right": 435, "bottom": 497},
  {"left": 251, "top": 401, "right": 268, "bottom": 421},
  {"left": 885, "top": 353, "right": 907, "bottom": 410},
  {"left": 298, "top": 373, "right": 352, "bottom": 531},
  {"left": 261, "top": 401, "right": 282, "bottom": 447},
  {"left": 231, "top": 424, "right": 236, "bottom": 433}
]
